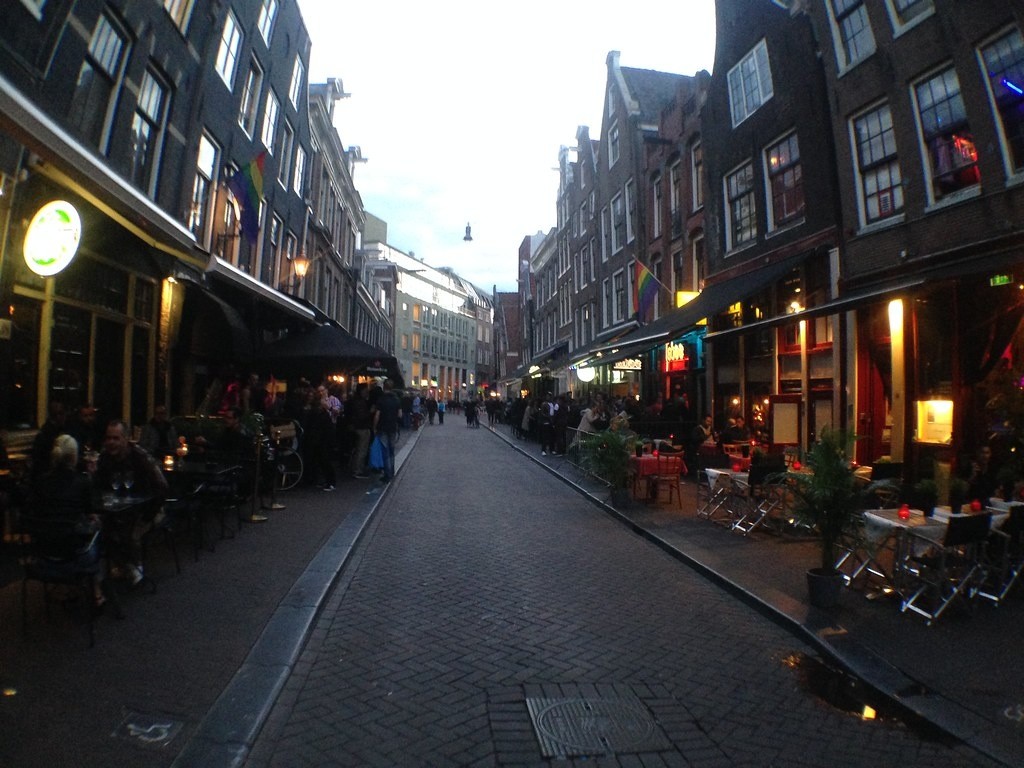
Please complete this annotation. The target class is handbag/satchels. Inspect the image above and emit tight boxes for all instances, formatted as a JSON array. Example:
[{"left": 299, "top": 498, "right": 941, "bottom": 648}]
[{"left": 367, "top": 435, "right": 384, "bottom": 469}]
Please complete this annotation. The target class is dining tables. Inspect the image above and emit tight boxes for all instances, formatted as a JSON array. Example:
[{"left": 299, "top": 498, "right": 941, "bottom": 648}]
[
  {"left": 629, "top": 453, "right": 688, "bottom": 505},
  {"left": 698, "top": 439, "right": 819, "bottom": 537},
  {"left": 835, "top": 496, "right": 1024, "bottom": 623}
]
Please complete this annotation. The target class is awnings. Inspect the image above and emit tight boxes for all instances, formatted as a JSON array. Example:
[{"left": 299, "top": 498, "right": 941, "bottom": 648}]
[
  {"left": 251, "top": 326, "right": 405, "bottom": 388},
  {"left": 513, "top": 243, "right": 952, "bottom": 380}
]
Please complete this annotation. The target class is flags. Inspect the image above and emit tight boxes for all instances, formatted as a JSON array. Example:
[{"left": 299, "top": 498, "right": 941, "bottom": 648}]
[{"left": 226, "top": 151, "right": 266, "bottom": 243}]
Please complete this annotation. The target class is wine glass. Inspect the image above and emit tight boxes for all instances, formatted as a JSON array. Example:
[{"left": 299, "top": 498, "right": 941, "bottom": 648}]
[
  {"left": 123, "top": 470, "right": 136, "bottom": 502},
  {"left": 110, "top": 472, "right": 122, "bottom": 503}
]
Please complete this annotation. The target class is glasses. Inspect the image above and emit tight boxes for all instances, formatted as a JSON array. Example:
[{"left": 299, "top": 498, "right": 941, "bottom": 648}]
[{"left": 223, "top": 415, "right": 235, "bottom": 421}]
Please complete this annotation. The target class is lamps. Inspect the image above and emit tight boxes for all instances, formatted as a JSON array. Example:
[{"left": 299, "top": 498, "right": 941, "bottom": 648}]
[{"left": 282, "top": 249, "right": 311, "bottom": 289}]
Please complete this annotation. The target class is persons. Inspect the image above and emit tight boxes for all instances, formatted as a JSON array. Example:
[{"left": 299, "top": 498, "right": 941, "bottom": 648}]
[
  {"left": 962, "top": 440, "right": 1012, "bottom": 504},
  {"left": 70, "top": 407, "right": 103, "bottom": 442},
  {"left": 220, "top": 410, "right": 250, "bottom": 451},
  {"left": 30, "top": 433, "right": 108, "bottom": 610},
  {"left": 229, "top": 372, "right": 751, "bottom": 495},
  {"left": 147, "top": 406, "right": 177, "bottom": 456},
  {"left": 93, "top": 419, "right": 168, "bottom": 593}
]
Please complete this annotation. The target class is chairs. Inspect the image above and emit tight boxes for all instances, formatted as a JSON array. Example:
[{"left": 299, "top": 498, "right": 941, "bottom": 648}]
[
  {"left": 648, "top": 449, "right": 683, "bottom": 509},
  {"left": 572, "top": 437, "right": 601, "bottom": 458},
  {"left": 1, "top": 436, "right": 254, "bottom": 657}
]
